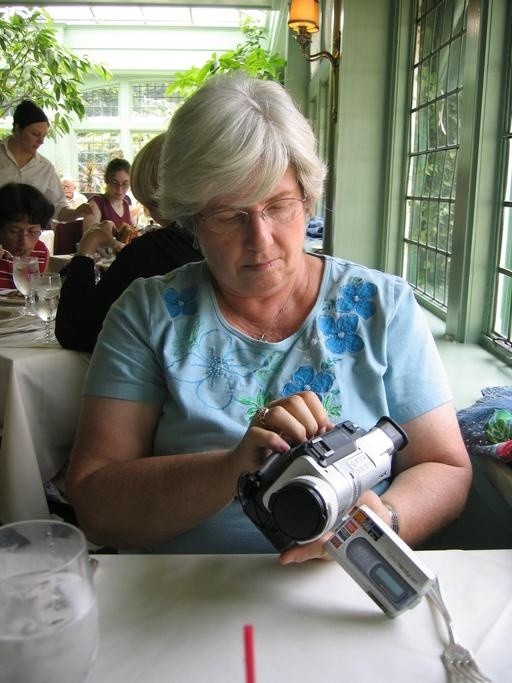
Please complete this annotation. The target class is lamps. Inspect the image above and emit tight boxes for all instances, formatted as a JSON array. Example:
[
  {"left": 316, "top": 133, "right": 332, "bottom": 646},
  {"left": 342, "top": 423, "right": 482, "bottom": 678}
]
[{"left": 287, "top": 0, "right": 338, "bottom": 62}]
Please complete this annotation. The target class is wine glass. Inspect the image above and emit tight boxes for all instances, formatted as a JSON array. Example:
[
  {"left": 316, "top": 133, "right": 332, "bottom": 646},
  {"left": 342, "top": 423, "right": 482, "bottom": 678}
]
[{"left": 12, "top": 254, "right": 64, "bottom": 346}]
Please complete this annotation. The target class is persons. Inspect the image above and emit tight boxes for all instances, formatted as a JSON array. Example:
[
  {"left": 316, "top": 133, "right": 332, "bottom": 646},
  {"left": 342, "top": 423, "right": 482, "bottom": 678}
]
[
  {"left": 0, "top": 181, "right": 56, "bottom": 291},
  {"left": 107, "top": 148, "right": 146, "bottom": 226},
  {"left": 55, "top": 130, "right": 204, "bottom": 354},
  {"left": 2, "top": 98, "right": 95, "bottom": 225},
  {"left": 81, "top": 157, "right": 139, "bottom": 236},
  {"left": 66, "top": 67, "right": 475, "bottom": 565},
  {"left": 49, "top": 174, "right": 88, "bottom": 233}
]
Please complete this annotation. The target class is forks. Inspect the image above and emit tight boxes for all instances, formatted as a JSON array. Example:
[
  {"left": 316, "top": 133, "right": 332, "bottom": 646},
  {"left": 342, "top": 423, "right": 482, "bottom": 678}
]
[{"left": 425, "top": 574, "right": 492, "bottom": 683}]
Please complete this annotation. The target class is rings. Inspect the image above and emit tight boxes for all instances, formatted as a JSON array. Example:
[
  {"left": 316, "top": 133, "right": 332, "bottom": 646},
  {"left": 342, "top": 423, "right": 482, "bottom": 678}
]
[{"left": 256, "top": 407, "right": 271, "bottom": 427}]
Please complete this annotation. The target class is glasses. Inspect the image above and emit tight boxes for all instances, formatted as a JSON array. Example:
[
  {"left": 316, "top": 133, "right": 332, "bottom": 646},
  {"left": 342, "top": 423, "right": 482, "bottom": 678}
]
[
  {"left": 6, "top": 229, "right": 41, "bottom": 240},
  {"left": 197, "top": 194, "right": 308, "bottom": 235}
]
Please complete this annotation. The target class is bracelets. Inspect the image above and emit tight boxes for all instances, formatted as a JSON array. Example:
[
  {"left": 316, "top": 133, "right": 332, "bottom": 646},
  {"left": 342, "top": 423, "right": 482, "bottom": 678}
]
[{"left": 382, "top": 499, "right": 401, "bottom": 537}]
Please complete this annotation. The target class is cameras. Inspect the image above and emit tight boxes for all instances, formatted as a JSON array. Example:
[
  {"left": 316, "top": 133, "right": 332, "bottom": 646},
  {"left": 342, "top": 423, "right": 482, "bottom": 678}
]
[{"left": 236, "top": 416, "right": 437, "bottom": 618}]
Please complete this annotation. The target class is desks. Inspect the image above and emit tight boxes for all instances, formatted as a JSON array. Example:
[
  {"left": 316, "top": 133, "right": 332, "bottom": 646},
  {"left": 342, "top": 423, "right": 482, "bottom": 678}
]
[{"left": 0, "top": 546, "right": 512, "bottom": 683}]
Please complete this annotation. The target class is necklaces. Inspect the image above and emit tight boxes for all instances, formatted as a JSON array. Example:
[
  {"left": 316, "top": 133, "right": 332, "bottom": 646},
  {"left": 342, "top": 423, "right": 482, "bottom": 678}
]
[
  {"left": 216, "top": 272, "right": 301, "bottom": 344},
  {"left": 109, "top": 195, "right": 123, "bottom": 216}
]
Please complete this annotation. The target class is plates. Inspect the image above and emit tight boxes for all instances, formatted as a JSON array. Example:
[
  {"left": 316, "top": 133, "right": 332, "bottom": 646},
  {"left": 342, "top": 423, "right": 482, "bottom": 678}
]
[{"left": 0, "top": 290, "right": 25, "bottom": 323}]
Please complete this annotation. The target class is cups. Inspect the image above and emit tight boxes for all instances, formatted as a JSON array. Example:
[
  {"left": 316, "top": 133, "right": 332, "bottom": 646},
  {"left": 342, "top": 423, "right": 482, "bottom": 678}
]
[{"left": 0, "top": 521, "right": 100, "bottom": 682}]
